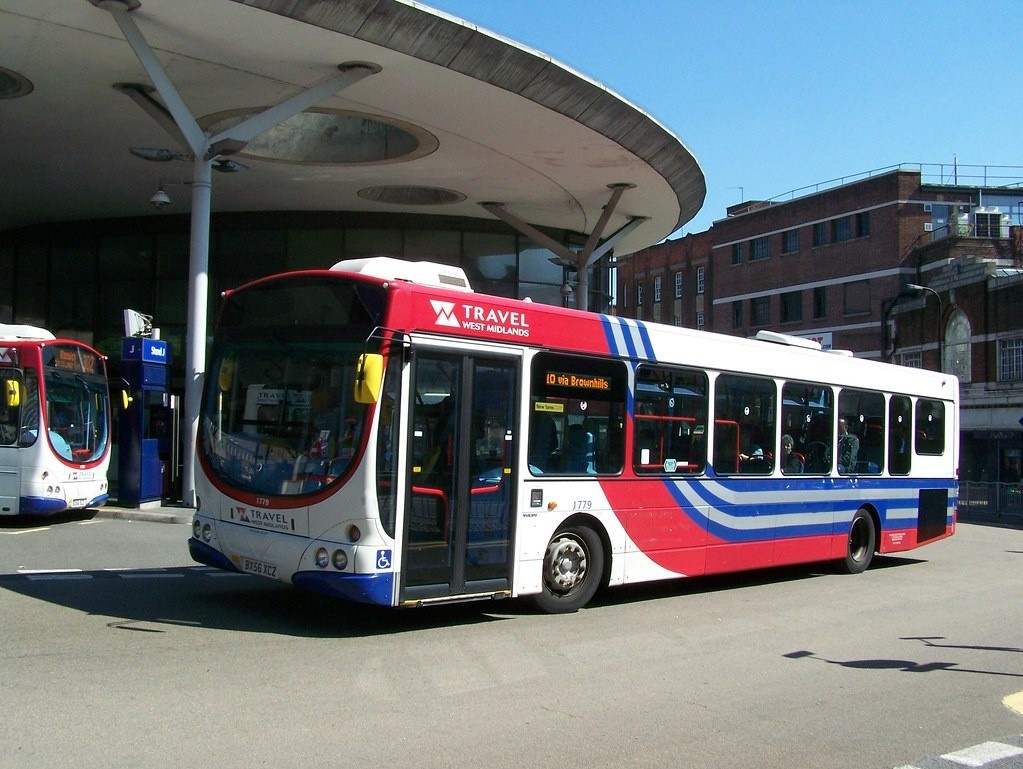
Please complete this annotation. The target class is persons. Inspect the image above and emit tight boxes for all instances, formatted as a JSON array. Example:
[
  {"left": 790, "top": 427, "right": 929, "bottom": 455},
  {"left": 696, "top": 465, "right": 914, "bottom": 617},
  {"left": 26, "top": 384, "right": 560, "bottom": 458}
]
[
  {"left": 728, "top": 426, "right": 763, "bottom": 460},
  {"left": 780, "top": 434, "right": 800, "bottom": 473},
  {"left": 827, "top": 415, "right": 859, "bottom": 473}
]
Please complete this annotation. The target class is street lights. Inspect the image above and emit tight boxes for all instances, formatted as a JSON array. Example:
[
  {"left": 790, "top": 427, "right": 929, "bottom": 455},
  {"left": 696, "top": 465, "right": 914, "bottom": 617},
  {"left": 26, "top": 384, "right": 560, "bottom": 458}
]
[{"left": 906, "top": 284, "right": 944, "bottom": 373}]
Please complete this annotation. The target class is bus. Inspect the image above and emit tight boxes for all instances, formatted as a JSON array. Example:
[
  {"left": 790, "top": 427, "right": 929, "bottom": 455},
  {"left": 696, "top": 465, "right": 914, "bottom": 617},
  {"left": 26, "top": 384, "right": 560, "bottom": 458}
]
[
  {"left": 0, "top": 322, "right": 113, "bottom": 524},
  {"left": 189, "top": 256, "right": 960, "bottom": 613}
]
